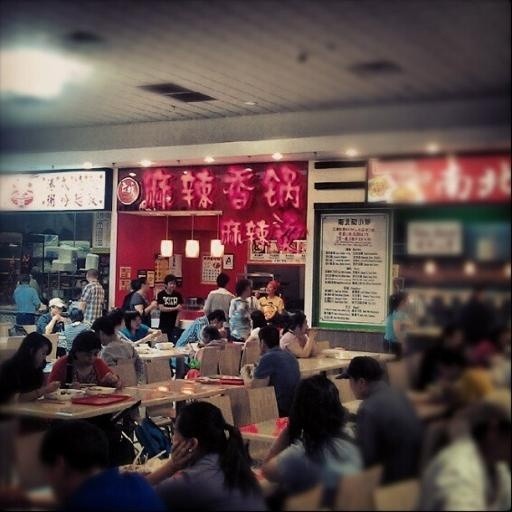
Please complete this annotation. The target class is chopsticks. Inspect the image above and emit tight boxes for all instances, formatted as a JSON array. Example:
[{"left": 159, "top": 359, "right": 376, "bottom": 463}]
[{"left": 66, "top": 383, "right": 97, "bottom": 388}]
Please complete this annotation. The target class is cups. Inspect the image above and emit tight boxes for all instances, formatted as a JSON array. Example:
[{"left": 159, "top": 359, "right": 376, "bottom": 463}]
[{"left": 139, "top": 343, "right": 148, "bottom": 348}]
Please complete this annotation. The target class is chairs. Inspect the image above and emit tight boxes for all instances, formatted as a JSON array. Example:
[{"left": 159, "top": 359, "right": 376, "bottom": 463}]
[
  {"left": 217, "top": 350, "right": 241, "bottom": 383},
  {"left": 145, "top": 356, "right": 182, "bottom": 423},
  {"left": 287, "top": 484, "right": 330, "bottom": 512},
  {"left": 373, "top": 478, "right": 425, "bottom": 512},
  {"left": 245, "top": 385, "right": 286, "bottom": 457},
  {"left": 328, "top": 373, "right": 361, "bottom": 418},
  {"left": 193, "top": 395, "right": 238, "bottom": 430},
  {"left": 335, "top": 464, "right": 383, "bottom": 512},
  {"left": 236, "top": 338, "right": 261, "bottom": 366},
  {"left": 189, "top": 345, "right": 219, "bottom": 380},
  {"left": 108, "top": 364, "right": 139, "bottom": 387},
  {"left": 226, "top": 342, "right": 244, "bottom": 374},
  {"left": 150, "top": 333, "right": 168, "bottom": 350}
]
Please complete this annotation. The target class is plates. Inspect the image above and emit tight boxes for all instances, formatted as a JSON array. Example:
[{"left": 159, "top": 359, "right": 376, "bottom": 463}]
[
  {"left": 72, "top": 393, "right": 132, "bottom": 406},
  {"left": 194, "top": 375, "right": 244, "bottom": 385}
]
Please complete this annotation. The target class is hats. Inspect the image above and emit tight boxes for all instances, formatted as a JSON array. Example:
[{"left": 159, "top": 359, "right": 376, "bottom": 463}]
[
  {"left": 334, "top": 356, "right": 381, "bottom": 381},
  {"left": 48, "top": 297, "right": 67, "bottom": 309}
]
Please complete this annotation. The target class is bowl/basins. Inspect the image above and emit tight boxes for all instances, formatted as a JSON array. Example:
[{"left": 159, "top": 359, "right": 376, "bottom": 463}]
[
  {"left": 155, "top": 342, "right": 173, "bottom": 350},
  {"left": 45, "top": 389, "right": 85, "bottom": 401},
  {"left": 82, "top": 385, "right": 117, "bottom": 395}
]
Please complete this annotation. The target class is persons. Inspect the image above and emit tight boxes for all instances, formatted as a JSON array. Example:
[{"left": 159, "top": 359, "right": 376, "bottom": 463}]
[
  {"left": 65, "top": 308, "right": 91, "bottom": 356},
  {"left": 107, "top": 309, "right": 138, "bottom": 348},
  {"left": 405, "top": 289, "right": 512, "bottom": 406},
  {"left": 13, "top": 273, "right": 41, "bottom": 326},
  {"left": 281, "top": 311, "right": 319, "bottom": 358},
  {"left": 383, "top": 291, "right": 412, "bottom": 361},
  {"left": 129, "top": 277, "right": 157, "bottom": 348},
  {"left": 258, "top": 281, "right": 284, "bottom": 323},
  {"left": 204, "top": 273, "right": 236, "bottom": 324},
  {"left": 189, "top": 325, "right": 221, "bottom": 369},
  {"left": 262, "top": 375, "right": 365, "bottom": 511},
  {"left": 227, "top": 278, "right": 258, "bottom": 342},
  {"left": 36, "top": 298, "right": 66, "bottom": 335},
  {"left": 417, "top": 391, "right": 512, "bottom": 512},
  {"left": 176, "top": 309, "right": 227, "bottom": 347},
  {"left": 39, "top": 419, "right": 165, "bottom": 512},
  {"left": 122, "top": 279, "right": 138, "bottom": 310},
  {"left": 80, "top": 269, "right": 105, "bottom": 325},
  {"left": 91, "top": 316, "right": 143, "bottom": 420},
  {"left": 245, "top": 310, "right": 272, "bottom": 343},
  {"left": 48, "top": 330, "right": 124, "bottom": 390},
  {"left": 240, "top": 327, "right": 301, "bottom": 417},
  {"left": 336, "top": 356, "right": 420, "bottom": 485},
  {"left": 143, "top": 402, "right": 268, "bottom": 511},
  {"left": 157, "top": 274, "right": 183, "bottom": 342},
  {"left": 120, "top": 311, "right": 162, "bottom": 346},
  {"left": 0, "top": 332, "right": 61, "bottom": 406}
]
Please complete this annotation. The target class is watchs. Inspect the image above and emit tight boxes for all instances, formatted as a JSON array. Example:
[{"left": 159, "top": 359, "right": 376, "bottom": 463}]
[{"left": 35, "top": 390, "right": 43, "bottom": 398}]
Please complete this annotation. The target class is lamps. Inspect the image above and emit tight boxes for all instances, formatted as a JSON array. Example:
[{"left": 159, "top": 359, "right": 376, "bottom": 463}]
[
  {"left": 162, "top": 215, "right": 174, "bottom": 257},
  {"left": 185, "top": 215, "right": 200, "bottom": 259},
  {"left": 210, "top": 214, "right": 225, "bottom": 257}
]
